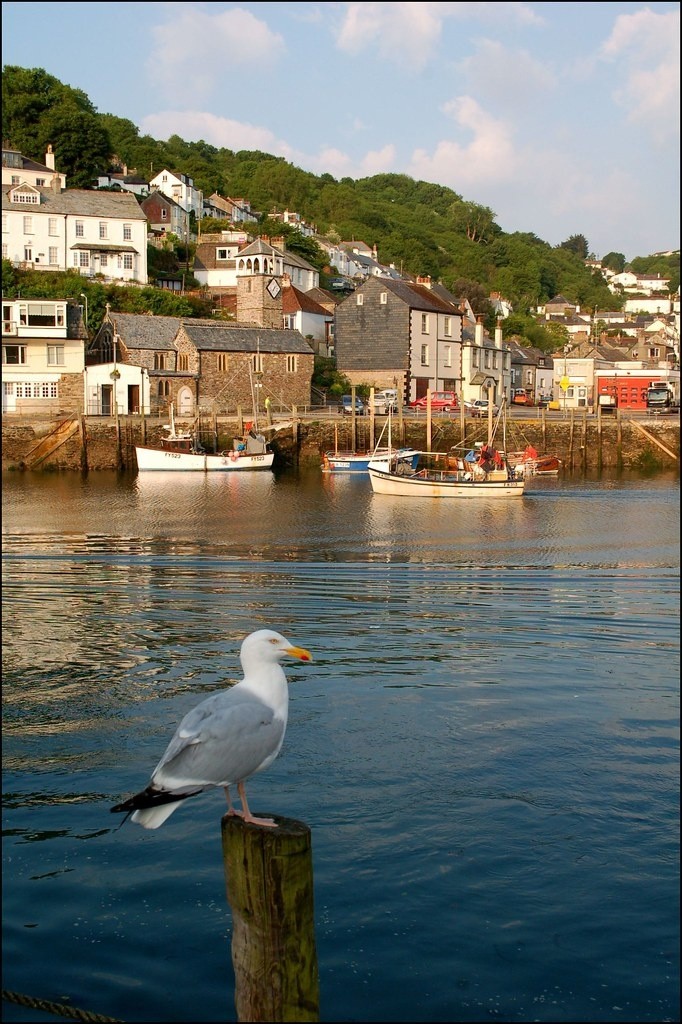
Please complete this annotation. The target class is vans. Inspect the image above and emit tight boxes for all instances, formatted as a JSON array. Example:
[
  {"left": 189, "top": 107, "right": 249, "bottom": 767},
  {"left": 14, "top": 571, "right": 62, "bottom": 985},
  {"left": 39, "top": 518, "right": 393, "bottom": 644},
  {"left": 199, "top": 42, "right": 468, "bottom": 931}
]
[{"left": 514, "top": 393, "right": 535, "bottom": 407}]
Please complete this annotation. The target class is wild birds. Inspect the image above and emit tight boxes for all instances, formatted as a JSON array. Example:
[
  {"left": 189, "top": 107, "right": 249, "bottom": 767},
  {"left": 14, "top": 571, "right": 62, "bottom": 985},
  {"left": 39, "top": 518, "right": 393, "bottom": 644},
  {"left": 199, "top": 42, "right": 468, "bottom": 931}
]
[{"left": 106, "top": 628, "right": 314, "bottom": 833}]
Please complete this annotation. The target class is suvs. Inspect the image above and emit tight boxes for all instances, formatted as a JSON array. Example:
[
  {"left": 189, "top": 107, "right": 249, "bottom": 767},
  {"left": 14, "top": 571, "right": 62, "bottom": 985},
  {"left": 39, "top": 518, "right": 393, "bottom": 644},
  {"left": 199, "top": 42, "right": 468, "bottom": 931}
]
[
  {"left": 366, "top": 389, "right": 399, "bottom": 415},
  {"left": 336, "top": 395, "right": 363, "bottom": 414},
  {"left": 410, "top": 390, "right": 472, "bottom": 413}
]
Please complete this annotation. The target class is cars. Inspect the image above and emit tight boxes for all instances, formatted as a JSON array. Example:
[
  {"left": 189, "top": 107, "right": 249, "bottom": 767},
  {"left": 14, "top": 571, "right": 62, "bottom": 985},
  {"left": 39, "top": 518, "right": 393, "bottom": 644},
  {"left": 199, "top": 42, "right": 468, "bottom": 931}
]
[
  {"left": 537, "top": 395, "right": 553, "bottom": 409},
  {"left": 470, "top": 399, "right": 500, "bottom": 417}
]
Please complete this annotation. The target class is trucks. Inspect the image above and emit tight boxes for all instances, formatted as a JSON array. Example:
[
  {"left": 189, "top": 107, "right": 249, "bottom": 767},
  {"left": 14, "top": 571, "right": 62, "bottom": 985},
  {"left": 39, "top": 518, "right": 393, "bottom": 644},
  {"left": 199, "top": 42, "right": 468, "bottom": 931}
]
[{"left": 642, "top": 380, "right": 680, "bottom": 415}]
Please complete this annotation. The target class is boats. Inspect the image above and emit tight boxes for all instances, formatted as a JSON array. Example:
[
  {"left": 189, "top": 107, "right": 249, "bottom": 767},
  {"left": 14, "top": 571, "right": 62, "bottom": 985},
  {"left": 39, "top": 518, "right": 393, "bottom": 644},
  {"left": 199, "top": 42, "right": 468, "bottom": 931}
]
[
  {"left": 135, "top": 403, "right": 274, "bottom": 471},
  {"left": 368, "top": 393, "right": 561, "bottom": 497},
  {"left": 321, "top": 448, "right": 422, "bottom": 474}
]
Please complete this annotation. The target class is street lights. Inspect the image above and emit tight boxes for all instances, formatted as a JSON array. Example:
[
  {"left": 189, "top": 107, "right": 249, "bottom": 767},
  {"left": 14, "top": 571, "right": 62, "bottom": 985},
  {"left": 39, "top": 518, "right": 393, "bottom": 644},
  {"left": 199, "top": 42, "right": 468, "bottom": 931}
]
[{"left": 81, "top": 293, "right": 88, "bottom": 331}]
